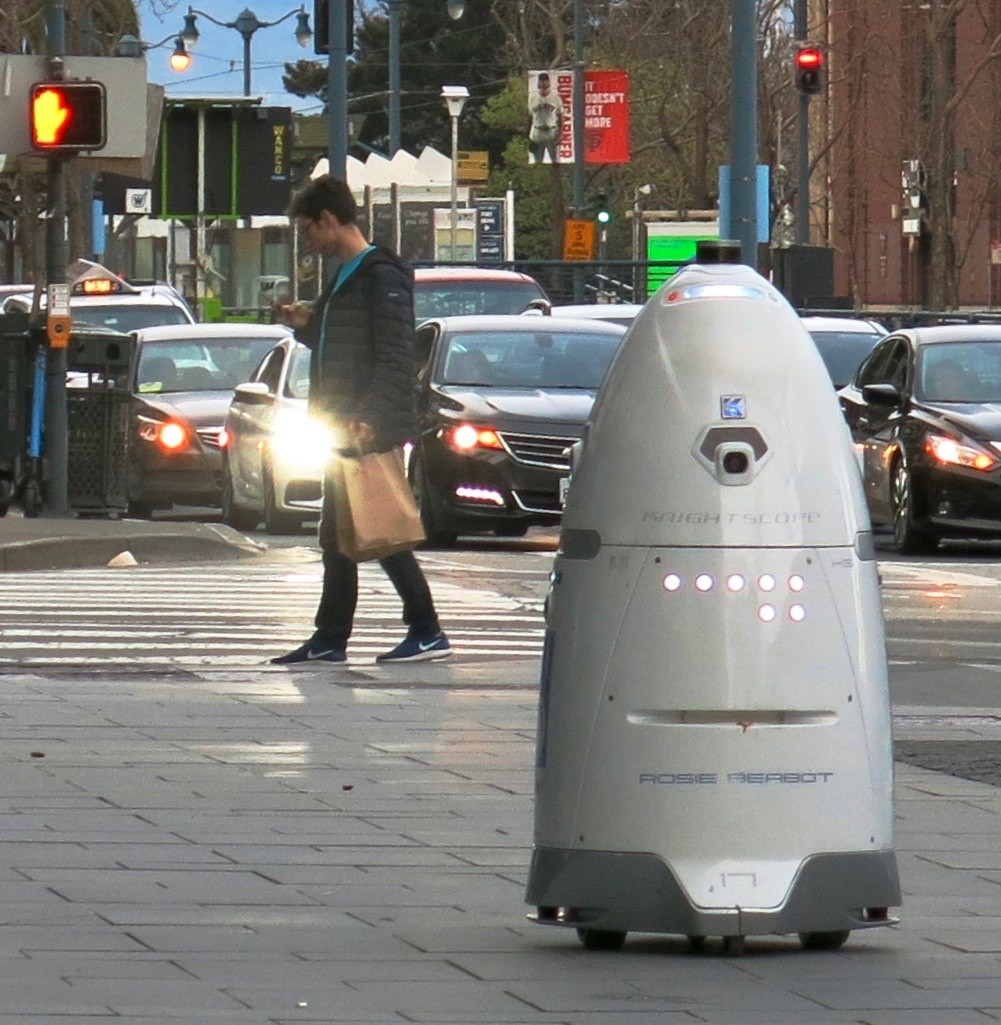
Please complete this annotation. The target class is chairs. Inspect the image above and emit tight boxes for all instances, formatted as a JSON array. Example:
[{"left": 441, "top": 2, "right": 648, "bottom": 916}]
[
  {"left": 140, "top": 357, "right": 179, "bottom": 391},
  {"left": 448, "top": 351, "right": 495, "bottom": 384},
  {"left": 183, "top": 368, "right": 214, "bottom": 390}
]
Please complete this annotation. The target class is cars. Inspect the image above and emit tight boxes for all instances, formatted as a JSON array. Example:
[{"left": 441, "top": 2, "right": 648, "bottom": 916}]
[
  {"left": 104, "top": 322, "right": 302, "bottom": 516},
  {"left": 414, "top": 267, "right": 562, "bottom": 316},
  {"left": 806, "top": 315, "right": 904, "bottom": 399},
  {"left": 0, "top": 259, "right": 220, "bottom": 513},
  {"left": 410, "top": 314, "right": 643, "bottom": 544},
  {"left": 225, "top": 336, "right": 415, "bottom": 535},
  {"left": 836, "top": 325, "right": 1001, "bottom": 553},
  {"left": 499, "top": 300, "right": 653, "bottom": 392}
]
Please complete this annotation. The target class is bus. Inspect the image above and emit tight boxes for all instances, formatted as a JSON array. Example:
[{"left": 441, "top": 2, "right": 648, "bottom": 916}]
[{"left": 193, "top": 219, "right": 305, "bottom": 326}]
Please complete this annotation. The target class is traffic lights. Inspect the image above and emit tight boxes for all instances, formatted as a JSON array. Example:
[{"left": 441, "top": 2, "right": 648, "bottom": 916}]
[
  {"left": 796, "top": 48, "right": 825, "bottom": 98},
  {"left": 27, "top": 79, "right": 109, "bottom": 151}
]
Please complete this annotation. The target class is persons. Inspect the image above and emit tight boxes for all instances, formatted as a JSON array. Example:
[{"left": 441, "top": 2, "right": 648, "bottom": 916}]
[
  {"left": 933, "top": 361, "right": 966, "bottom": 400},
  {"left": 270, "top": 173, "right": 452, "bottom": 664},
  {"left": 528, "top": 72, "right": 564, "bottom": 165}
]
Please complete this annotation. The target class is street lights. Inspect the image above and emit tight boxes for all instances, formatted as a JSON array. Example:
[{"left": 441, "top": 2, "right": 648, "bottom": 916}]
[
  {"left": 186, "top": 7, "right": 314, "bottom": 98},
  {"left": 441, "top": 86, "right": 476, "bottom": 263}
]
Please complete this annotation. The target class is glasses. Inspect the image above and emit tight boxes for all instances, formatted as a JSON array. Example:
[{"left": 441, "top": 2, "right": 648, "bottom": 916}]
[{"left": 297, "top": 215, "right": 318, "bottom": 238}]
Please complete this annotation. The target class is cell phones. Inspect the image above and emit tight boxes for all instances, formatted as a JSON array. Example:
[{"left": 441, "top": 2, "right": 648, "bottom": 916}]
[{"left": 261, "top": 293, "right": 283, "bottom": 311}]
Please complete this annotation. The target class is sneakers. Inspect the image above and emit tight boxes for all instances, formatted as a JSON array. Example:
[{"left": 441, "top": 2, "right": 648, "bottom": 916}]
[
  {"left": 376, "top": 633, "right": 452, "bottom": 664},
  {"left": 270, "top": 635, "right": 347, "bottom": 664}
]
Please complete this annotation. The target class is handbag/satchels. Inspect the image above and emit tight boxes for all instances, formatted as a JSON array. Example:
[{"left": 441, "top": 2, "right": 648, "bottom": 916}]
[{"left": 332, "top": 436, "right": 427, "bottom": 562}]
